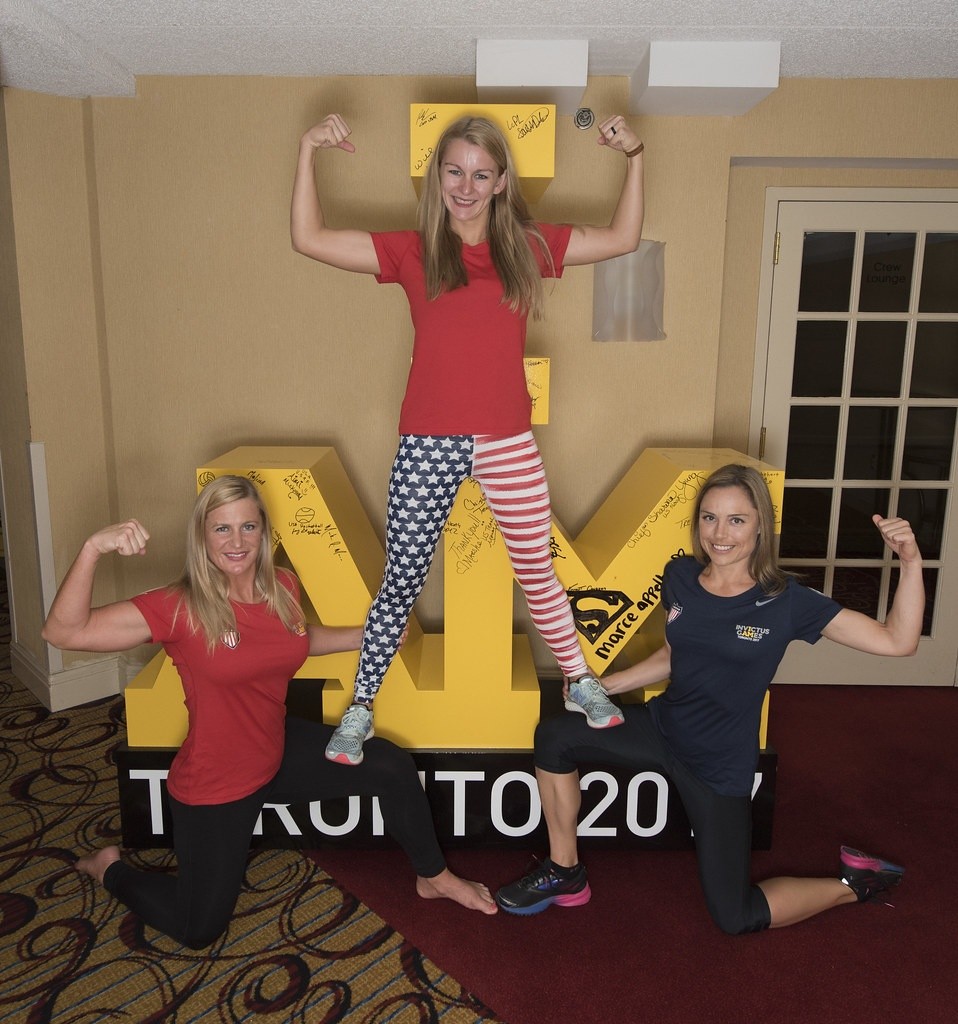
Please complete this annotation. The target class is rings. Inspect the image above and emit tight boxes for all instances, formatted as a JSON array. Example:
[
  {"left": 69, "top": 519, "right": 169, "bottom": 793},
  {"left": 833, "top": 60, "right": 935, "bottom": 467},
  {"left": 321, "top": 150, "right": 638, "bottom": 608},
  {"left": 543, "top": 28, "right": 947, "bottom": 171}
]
[{"left": 611, "top": 127, "right": 616, "bottom": 133}]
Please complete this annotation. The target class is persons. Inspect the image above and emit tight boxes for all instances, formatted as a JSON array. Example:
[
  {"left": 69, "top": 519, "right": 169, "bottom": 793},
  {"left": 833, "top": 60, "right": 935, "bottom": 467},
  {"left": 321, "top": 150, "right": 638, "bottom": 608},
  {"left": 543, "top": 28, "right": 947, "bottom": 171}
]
[
  {"left": 42, "top": 476, "right": 497, "bottom": 950},
  {"left": 498, "top": 464, "right": 925, "bottom": 936},
  {"left": 290, "top": 115, "right": 647, "bottom": 765}
]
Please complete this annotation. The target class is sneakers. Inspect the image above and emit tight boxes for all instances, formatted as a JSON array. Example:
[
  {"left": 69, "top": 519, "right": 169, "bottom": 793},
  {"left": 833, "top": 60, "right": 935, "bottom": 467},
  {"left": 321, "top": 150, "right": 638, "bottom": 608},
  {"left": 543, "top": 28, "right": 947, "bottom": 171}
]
[
  {"left": 565, "top": 674, "right": 625, "bottom": 729},
  {"left": 494, "top": 854, "right": 592, "bottom": 916},
  {"left": 325, "top": 704, "right": 375, "bottom": 765},
  {"left": 838, "top": 846, "right": 905, "bottom": 902}
]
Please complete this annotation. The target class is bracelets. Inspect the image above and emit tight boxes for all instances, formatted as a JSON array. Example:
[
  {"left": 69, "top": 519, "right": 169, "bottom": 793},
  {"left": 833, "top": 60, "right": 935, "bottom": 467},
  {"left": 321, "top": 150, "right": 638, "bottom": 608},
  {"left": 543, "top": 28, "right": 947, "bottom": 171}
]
[{"left": 625, "top": 143, "right": 644, "bottom": 158}]
